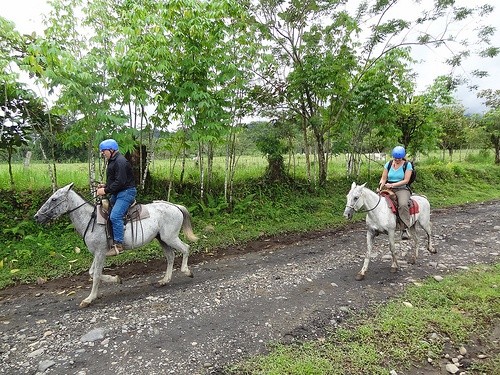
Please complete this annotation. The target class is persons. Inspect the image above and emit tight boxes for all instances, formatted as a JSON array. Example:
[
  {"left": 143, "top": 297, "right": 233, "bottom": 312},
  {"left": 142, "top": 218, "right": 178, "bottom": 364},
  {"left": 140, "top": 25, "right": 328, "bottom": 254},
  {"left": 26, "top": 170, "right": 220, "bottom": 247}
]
[
  {"left": 376, "top": 146, "right": 412, "bottom": 240},
  {"left": 97, "top": 139, "right": 137, "bottom": 256}
]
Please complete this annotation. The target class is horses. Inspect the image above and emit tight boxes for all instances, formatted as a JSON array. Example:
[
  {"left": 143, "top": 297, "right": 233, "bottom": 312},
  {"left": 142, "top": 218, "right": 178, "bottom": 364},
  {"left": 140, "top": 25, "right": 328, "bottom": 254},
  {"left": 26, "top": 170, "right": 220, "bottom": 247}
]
[
  {"left": 33, "top": 181, "right": 200, "bottom": 309},
  {"left": 343, "top": 182, "right": 438, "bottom": 281}
]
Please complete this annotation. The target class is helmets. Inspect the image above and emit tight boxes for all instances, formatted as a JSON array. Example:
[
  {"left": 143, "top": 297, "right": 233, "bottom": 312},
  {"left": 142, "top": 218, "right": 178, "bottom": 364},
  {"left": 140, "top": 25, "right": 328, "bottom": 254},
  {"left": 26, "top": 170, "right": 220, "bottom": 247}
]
[
  {"left": 392, "top": 146, "right": 406, "bottom": 159},
  {"left": 99, "top": 139, "right": 118, "bottom": 151}
]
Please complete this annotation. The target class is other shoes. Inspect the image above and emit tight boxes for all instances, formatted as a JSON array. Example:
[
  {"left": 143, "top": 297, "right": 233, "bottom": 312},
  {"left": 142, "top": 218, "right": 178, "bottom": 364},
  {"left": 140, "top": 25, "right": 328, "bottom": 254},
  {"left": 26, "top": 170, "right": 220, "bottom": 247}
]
[
  {"left": 401, "top": 232, "right": 409, "bottom": 239},
  {"left": 105, "top": 247, "right": 124, "bottom": 255}
]
